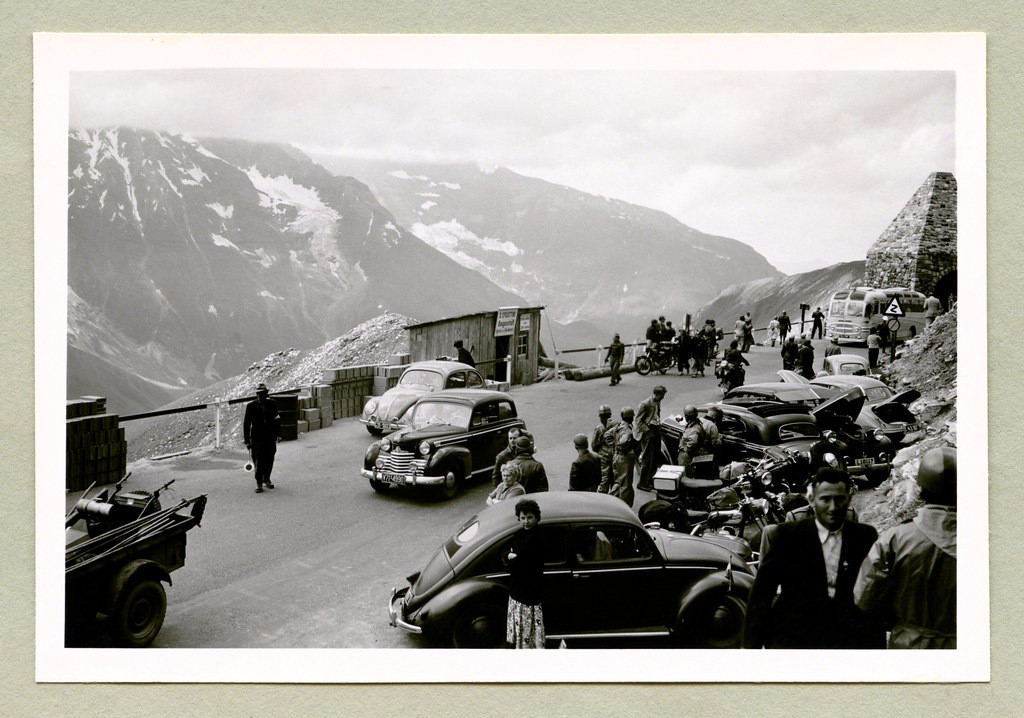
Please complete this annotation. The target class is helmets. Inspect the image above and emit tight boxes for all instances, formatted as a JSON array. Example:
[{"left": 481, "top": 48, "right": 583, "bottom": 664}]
[
  {"left": 621, "top": 407, "right": 635, "bottom": 417},
  {"left": 599, "top": 404, "right": 611, "bottom": 414},
  {"left": 917, "top": 447, "right": 957, "bottom": 489},
  {"left": 684, "top": 404, "right": 698, "bottom": 416}
]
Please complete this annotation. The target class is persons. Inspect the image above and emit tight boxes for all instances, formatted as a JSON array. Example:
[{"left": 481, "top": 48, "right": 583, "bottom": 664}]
[
  {"left": 877, "top": 316, "right": 890, "bottom": 354},
  {"left": 854, "top": 447, "right": 958, "bottom": 649},
  {"left": 508, "top": 437, "right": 549, "bottom": 492},
  {"left": 604, "top": 333, "right": 624, "bottom": 385},
  {"left": 646, "top": 303, "right": 828, "bottom": 395},
  {"left": 867, "top": 327, "right": 882, "bottom": 369},
  {"left": 486, "top": 462, "right": 525, "bottom": 506},
  {"left": 454, "top": 339, "right": 477, "bottom": 368},
  {"left": 504, "top": 499, "right": 547, "bottom": 649},
  {"left": 923, "top": 292, "right": 942, "bottom": 327},
  {"left": 243, "top": 384, "right": 281, "bottom": 493},
  {"left": 492, "top": 427, "right": 523, "bottom": 486},
  {"left": 568, "top": 385, "right": 721, "bottom": 507},
  {"left": 746, "top": 468, "right": 888, "bottom": 649},
  {"left": 824, "top": 336, "right": 842, "bottom": 357}
]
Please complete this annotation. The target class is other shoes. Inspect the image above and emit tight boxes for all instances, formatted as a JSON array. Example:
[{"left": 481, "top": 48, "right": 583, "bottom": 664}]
[
  {"left": 255, "top": 484, "right": 263, "bottom": 493},
  {"left": 616, "top": 377, "right": 622, "bottom": 384},
  {"left": 637, "top": 483, "right": 654, "bottom": 492},
  {"left": 266, "top": 480, "right": 274, "bottom": 489},
  {"left": 609, "top": 383, "right": 616, "bottom": 386}
]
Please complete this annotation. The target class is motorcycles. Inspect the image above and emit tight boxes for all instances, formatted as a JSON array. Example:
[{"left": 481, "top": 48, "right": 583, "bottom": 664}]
[
  {"left": 635, "top": 336, "right": 678, "bottom": 376},
  {"left": 640, "top": 447, "right": 794, "bottom": 550},
  {"left": 719, "top": 361, "right": 745, "bottom": 396}
]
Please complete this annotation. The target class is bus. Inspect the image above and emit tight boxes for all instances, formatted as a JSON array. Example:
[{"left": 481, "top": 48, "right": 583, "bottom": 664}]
[{"left": 826, "top": 284, "right": 928, "bottom": 347}]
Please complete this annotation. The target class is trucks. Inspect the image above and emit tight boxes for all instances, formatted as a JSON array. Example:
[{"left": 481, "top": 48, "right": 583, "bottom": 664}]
[{"left": 65, "top": 470, "right": 208, "bottom": 647}]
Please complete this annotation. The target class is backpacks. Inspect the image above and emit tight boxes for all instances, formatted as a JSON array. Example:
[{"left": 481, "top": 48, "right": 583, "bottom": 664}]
[{"left": 706, "top": 488, "right": 739, "bottom": 512}]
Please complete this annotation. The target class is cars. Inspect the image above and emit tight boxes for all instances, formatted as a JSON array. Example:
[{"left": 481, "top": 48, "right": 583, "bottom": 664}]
[
  {"left": 388, "top": 488, "right": 760, "bottom": 649},
  {"left": 359, "top": 388, "right": 542, "bottom": 499},
  {"left": 659, "top": 370, "right": 922, "bottom": 492},
  {"left": 824, "top": 355, "right": 873, "bottom": 378}
]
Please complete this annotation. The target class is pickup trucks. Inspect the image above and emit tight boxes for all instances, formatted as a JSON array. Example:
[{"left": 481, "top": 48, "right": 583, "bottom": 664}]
[{"left": 357, "top": 359, "right": 511, "bottom": 436}]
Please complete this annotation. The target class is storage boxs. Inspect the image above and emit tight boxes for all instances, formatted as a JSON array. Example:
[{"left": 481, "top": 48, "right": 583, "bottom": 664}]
[
  {"left": 689, "top": 454, "right": 717, "bottom": 477},
  {"left": 639, "top": 500, "right": 672, "bottom": 525}
]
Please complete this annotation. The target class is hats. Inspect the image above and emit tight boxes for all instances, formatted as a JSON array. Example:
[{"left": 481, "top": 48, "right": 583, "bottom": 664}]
[
  {"left": 516, "top": 436, "right": 530, "bottom": 450},
  {"left": 255, "top": 383, "right": 269, "bottom": 393},
  {"left": 801, "top": 333, "right": 806, "bottom": 338},
  {"left": 573, "top": 434, "right": 588, "bottom": 446},
  {"left": 832, "top": 337, "right": 838, "bottom": 342},
  {"left": 882, "top": 316, "right": 888, "bottom": 321},
  {"left": 454, "top": 340, "right": 463, "bottom": 348}
]
